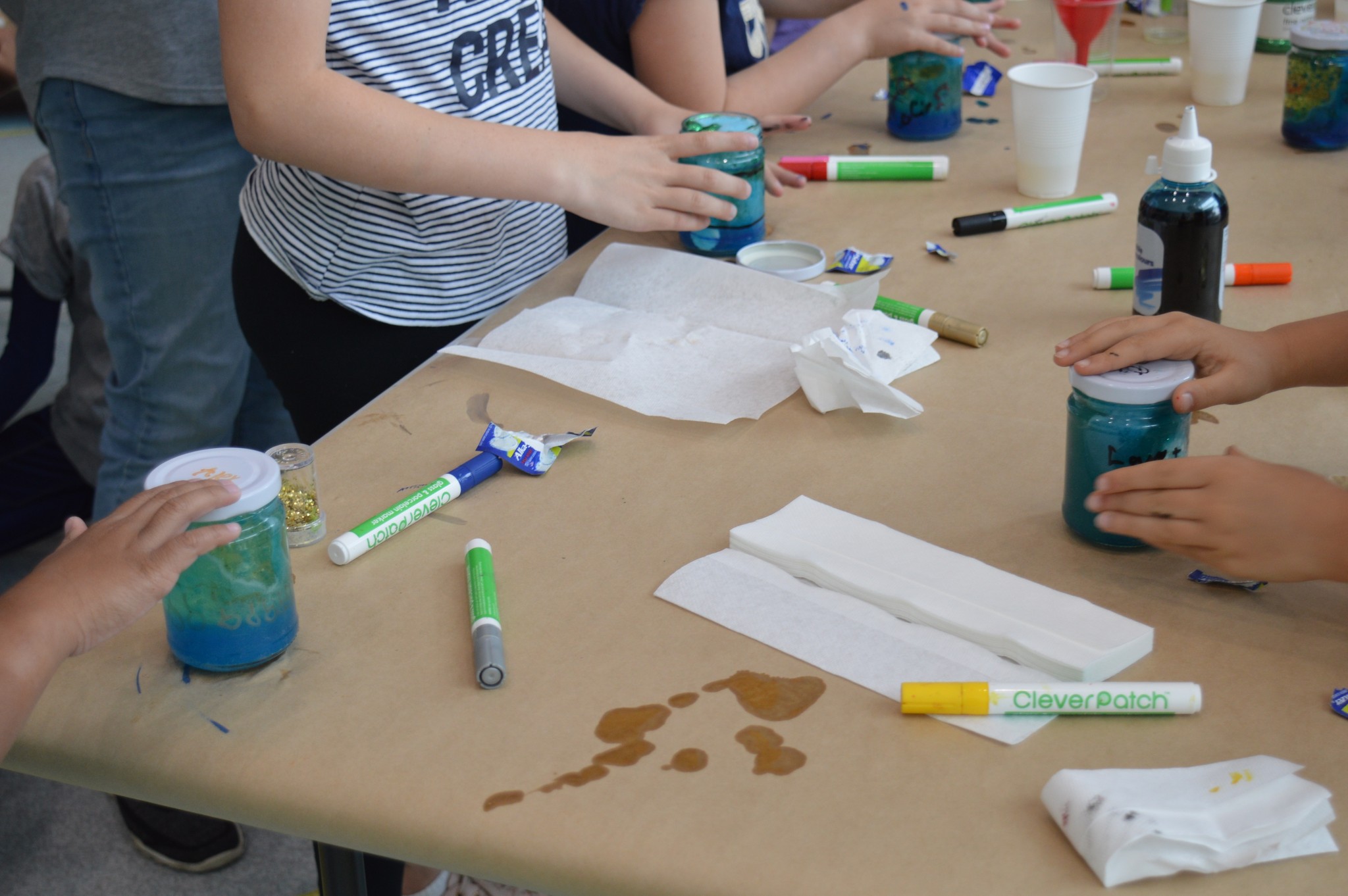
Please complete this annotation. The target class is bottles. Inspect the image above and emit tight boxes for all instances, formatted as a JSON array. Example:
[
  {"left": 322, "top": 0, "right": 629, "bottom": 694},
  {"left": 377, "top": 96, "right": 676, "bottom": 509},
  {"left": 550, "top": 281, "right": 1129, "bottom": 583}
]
[
  {"left": 1132, "top": 105, "right": 1231, "bottom": 325},
  {"left": 142, "top": 446, "right": 301, "bottom": 672},
  {"left": 677, "top": 110, "right": 768, "bottom": 262},
  {"left": 885, "top": 33, "right": 962, "bottom": 143},
  {"left": 1255, "top": 0, "right": 1315, "bottom": 52},
  {"left": 1061, "top": 356, "right": 1196, "bottom": 552},
  {"left": 1282, "top": 21, "right": 1348, "bottom": 152}
]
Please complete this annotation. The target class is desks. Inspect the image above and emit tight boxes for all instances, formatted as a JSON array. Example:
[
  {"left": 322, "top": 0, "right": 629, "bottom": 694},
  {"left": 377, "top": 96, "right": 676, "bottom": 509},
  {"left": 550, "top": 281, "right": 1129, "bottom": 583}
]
[{"left": 0, "top": 0, "right": 1348, "bottom": 896}]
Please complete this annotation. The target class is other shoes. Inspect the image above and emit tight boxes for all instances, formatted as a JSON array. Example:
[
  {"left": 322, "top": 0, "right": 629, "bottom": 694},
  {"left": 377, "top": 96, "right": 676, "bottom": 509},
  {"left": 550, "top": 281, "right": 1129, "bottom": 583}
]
[{"left": 113, "top": 787, "right": 246, "bottom": 874}]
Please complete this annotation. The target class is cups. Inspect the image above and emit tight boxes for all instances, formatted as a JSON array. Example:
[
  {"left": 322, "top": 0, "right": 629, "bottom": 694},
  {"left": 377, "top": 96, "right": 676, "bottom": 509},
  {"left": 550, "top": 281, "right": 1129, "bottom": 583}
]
[
  {"left": 1185, "top": 0, "right": 1265, "bottom": 107},
  {"left": 1050, "top": 0, "right": 1126, "bottom": 102},
  {"left": 1141, "top": 0, "right": 1188, "bottom": 43},
  {"left": 1006, "top": 62, "right": 1099, "bottom": 201}
]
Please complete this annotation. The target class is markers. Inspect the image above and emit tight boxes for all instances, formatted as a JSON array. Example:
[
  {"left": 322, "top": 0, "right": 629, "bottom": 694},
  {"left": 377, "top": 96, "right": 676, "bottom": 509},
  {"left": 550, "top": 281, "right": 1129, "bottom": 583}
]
[
  {"left": 328, "top": 452, "right": 504, "bottom": 565},
  {"left": 903, "top": 681, "right": 1201, "bottom": 719},
  {"left": 823, "top": 281, "right": 988, "bottom": 349},
  {"left": 953, "top": 192, "right": 1119, "bottom": 236},
  {"left": 1088, "top": 57, "right": 1181, "bottom": 77},
  {"left": 778, "top": 155, "right": 950, "bottom": 181},
  {"left": 465, "top": 538, "right": 504, "bottom": 689},
  {"left": 1094, "top": 262, "right": 1291, "bottom": 289}
]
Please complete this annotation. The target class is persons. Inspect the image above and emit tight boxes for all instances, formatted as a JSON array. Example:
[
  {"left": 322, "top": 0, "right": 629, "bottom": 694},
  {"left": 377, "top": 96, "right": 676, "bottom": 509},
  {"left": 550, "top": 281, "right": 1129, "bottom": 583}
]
[
  {"left": 1052, "top": 310, "right": 1347, "bottom": 582},
  {"left": 0, "top": 0, "right": 1020, "bottom": 873},
  {"left": 212, "top": 2, "right": 812, "bottom": 446}
]
